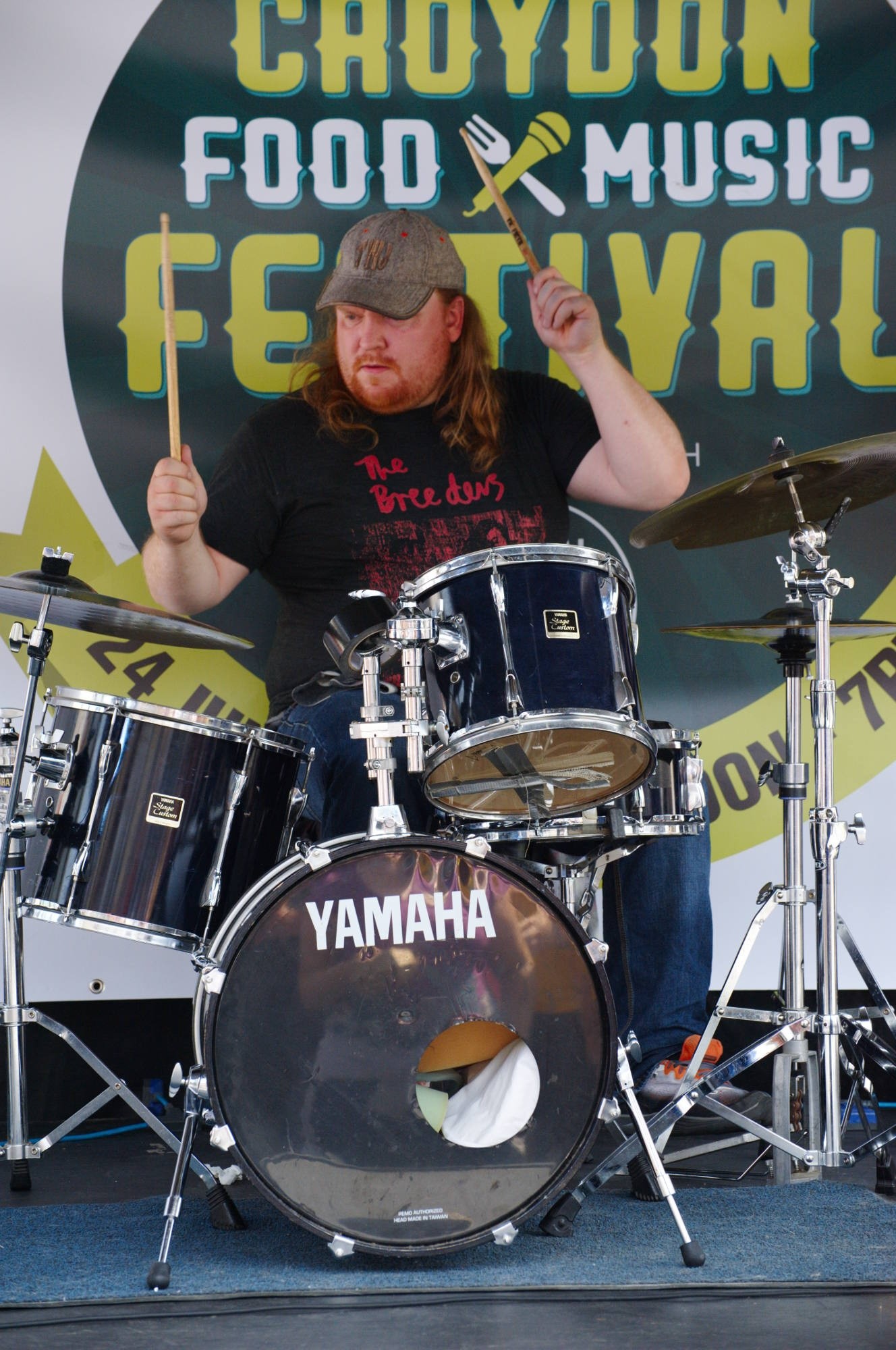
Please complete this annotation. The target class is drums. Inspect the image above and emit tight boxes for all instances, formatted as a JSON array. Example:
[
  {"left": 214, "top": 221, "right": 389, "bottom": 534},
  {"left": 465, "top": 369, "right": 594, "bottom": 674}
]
[
  {"left": 445, "top": 723, "right": 706, "bottom": 840},
  {"left": 394, "top": 537, "right": 658, "bottom": 822},
  {"left": 19, "top": 687, "right": 308, "bottom": 954},
  {"left": 195, "top": 831, "right": 620, "bottom": 1258}
]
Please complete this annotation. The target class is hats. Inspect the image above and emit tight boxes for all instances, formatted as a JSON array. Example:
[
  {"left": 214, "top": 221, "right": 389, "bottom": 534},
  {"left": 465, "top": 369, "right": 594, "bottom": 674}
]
[{"left": 314, "top": 206, "right": 468, "bottom": 320}]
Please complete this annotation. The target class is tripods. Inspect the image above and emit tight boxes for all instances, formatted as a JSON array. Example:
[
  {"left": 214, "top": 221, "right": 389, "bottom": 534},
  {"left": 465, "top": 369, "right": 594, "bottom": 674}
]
[{"left": 536, "top": 523, "right": 895, "bottom": 1241}]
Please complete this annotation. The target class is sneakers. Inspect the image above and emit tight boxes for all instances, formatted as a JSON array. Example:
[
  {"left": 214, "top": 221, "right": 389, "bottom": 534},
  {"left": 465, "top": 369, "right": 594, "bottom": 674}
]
[{"left": 598, "top": 1033, "right": 777, "bottom": 1137}]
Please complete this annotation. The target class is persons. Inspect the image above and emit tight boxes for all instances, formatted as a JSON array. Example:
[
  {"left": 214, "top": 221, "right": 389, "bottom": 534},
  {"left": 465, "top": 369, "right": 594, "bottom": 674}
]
[{"left": 142, "top": 206, "right": 751, "bottom": 1112}]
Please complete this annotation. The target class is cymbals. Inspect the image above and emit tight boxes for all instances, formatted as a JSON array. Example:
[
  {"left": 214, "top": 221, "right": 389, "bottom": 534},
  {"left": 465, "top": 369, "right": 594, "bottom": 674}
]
[
  {"left": 628, "top": 431, "right": 896, "bottom": 552},
  {"left": 659, "top": 608, "right": 896, "bottom": 645},
  {"left": 0, "top": 572, "right": 255, "bottom": 653}
]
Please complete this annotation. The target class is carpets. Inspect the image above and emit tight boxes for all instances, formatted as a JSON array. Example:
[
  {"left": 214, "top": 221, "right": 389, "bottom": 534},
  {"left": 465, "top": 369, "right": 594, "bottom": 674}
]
[{"left": 0, "top": 1181, "right": 896, "bottom": 1304}]
